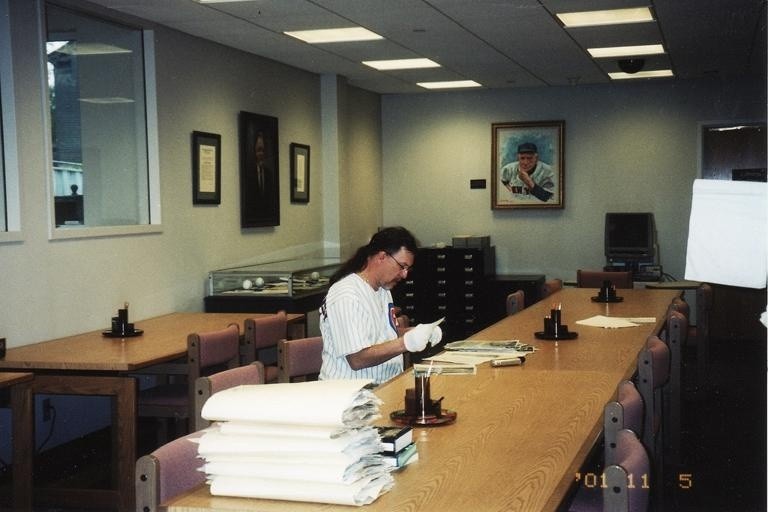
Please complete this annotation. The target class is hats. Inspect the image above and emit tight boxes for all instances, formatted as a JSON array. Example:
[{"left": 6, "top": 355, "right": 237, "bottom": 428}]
[{"left": 518, "top": 144, "right": 536, "bottom": 153}]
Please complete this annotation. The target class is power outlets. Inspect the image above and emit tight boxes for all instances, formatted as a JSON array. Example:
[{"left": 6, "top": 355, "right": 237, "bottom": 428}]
[{"left": 42, "top": 398, "right": 51, "bottom": 421}]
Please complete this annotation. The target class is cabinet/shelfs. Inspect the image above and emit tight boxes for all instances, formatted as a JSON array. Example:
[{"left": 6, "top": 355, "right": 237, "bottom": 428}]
[
  {"left": 205, "top": 255, "right": 350, "bottom": 340},
  {"left": 388, "top": 246, "right": 496, "bottom": 329}
]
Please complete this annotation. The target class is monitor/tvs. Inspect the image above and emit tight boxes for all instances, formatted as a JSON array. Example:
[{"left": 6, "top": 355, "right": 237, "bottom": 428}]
[{"left": 604, "top": 212, "right": 659, "bottom": 266}]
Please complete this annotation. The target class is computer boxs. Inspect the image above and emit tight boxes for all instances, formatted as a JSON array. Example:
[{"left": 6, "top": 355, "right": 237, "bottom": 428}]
[{"left": 603, "top": 265, "right": 662, "bottom": 281}]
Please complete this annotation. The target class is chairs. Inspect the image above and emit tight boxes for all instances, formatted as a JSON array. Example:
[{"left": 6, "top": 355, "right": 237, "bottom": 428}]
[
  {"left": 277, "top": 335, "right": 324, "bottom": 384},
  {"left": 543, "top": 277, "right": 563, "bottom": 295},
  {"left": 133, "top": 424, "right": 212, "bottom": 511},
  {"left": 395, "top": 313, "right": 411, "bottom": 340},
  {"left": 193, "top": 359, "right": 268, "bottom": 431},
  {"left": 138, "top": 322, "right": 241, "bottom": 455},
  {"left": 559, "top": 286, "right": 718, "bottom": 511},
  {"left": 506, "top": 290, "right": 525, "bottom": 314},
  {"left": 575, "top": 268, "right": 634, "bottom": 289},
  {"left": 394, "top": 306, "right": 400, "bottom": 316},
  {"left": 241, "top": 310, "right": 287, "bottom": 382}
]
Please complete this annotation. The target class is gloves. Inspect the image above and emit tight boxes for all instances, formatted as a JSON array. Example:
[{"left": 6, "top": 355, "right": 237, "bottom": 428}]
[{"left": 404, "top": 324, "right": 442, "bottom": 353}]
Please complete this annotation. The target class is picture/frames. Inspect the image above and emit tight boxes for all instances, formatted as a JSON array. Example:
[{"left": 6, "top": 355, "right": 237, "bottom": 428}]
[
  {"left": 490, "top": 119, "right": 567, "bottom": 211},
  {"left": 289, "top": 141, "right": 311, "bottom": 205},
  {"left": 236, "top": 111, "right": 281, "bottom": 228},
  {"left": 189, "top": 129, "right": 223, "bottom": 207}
]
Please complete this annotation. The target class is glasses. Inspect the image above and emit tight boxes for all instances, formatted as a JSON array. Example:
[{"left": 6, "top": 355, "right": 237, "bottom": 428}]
[{"left": 387, "top": 251, "right": 415, "bottom": 272}]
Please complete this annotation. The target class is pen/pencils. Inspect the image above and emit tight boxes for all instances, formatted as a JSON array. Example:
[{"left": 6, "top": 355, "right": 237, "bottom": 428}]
[
  {"left": 434, "top": 397, "right": 444, "bottom": 404},
  {"left": 125, "top": 301, "right": 129, "bottom": 310},
  {"left": 545, "top": 302, "right": 562, "bottom": 319},
  {"left": 426, "top": 361, "right": 434, "bottom": 378}
]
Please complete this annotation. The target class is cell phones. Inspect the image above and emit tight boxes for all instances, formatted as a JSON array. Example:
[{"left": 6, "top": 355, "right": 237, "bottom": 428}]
[{"left": 491, "top": 357, "right": 522, "bottom": 367}]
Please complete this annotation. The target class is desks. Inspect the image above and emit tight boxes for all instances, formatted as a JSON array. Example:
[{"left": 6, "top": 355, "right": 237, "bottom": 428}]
[
  {"left": 0, "top": 308, "right": 289, "bottom": 512},
  {"left": 163, "top": 287, "right": 681, "bottom": 510},
  {"left": 0, "top": 370, "right": 36, "bottom": 512}
]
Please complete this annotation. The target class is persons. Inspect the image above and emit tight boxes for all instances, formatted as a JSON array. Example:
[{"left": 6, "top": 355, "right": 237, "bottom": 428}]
[
  {"left": 318, "top": 226, "right": 443, "bottom": 382},
  {"left": 500, "top": 143, "right": 555, "bottom": 202}
]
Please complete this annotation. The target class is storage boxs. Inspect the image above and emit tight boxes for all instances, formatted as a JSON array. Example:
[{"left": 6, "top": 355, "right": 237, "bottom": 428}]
[{"left": 483, "top": 271, "right": 546, "bottom": 318}]
